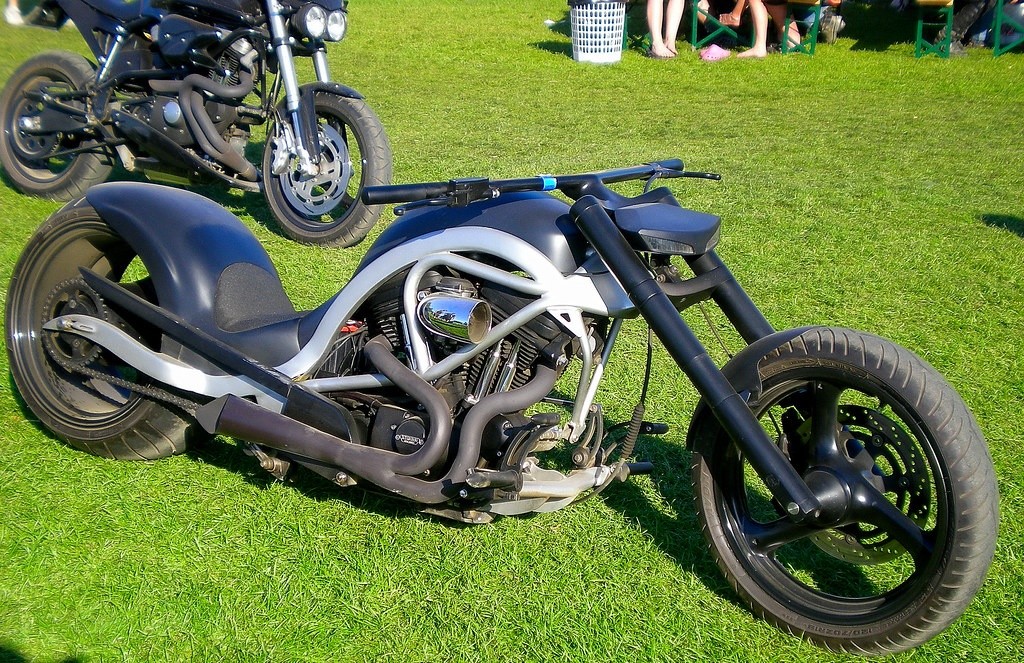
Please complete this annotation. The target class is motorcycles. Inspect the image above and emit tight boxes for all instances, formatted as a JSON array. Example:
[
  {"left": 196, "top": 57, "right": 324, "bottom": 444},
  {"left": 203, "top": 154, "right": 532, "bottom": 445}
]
[
  {"left": 0, "top": 0, "right": 393, "bottom": 249},
  {"left": 4, "top": 158, "right": 1001, "bottom": 660}
]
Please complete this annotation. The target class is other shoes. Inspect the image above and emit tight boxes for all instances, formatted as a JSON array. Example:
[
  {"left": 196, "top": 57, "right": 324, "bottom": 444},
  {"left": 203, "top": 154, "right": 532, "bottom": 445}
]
[
  {"left": 4, "top": 7, "right": 23, "bottom": 24},
  {"left": 821, "top": 15, "right": 845, "bottom": 44},
  {"left": 934, "top": 40, "right": 969, "bottom": 56}
]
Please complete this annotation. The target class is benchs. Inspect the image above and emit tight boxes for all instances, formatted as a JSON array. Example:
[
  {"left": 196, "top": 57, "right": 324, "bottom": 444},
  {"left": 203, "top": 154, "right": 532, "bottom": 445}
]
[
  {"left": 782, "top": 0, "right": 822, "bottom": 54},
  {"left": 915, "top": 0, "right": 955, "bottom": 58}
]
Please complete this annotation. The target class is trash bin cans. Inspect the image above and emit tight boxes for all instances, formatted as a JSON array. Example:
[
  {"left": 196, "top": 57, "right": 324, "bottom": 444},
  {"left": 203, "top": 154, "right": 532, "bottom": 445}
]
[{"left": 570, "top": 2, "right": 627, "bottom": 63}]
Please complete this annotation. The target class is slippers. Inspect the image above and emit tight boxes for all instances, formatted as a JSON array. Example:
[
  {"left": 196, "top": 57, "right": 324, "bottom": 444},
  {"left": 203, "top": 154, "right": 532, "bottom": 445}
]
[{"left": 700, "top": 44, "right": 730, "bottom": 62}]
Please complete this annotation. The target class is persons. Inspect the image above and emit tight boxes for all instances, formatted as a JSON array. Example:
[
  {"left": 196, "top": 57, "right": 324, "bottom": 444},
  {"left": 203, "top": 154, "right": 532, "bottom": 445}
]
[{"left": 643, "top": 0, "right": 1024, "bottom": 64}]
